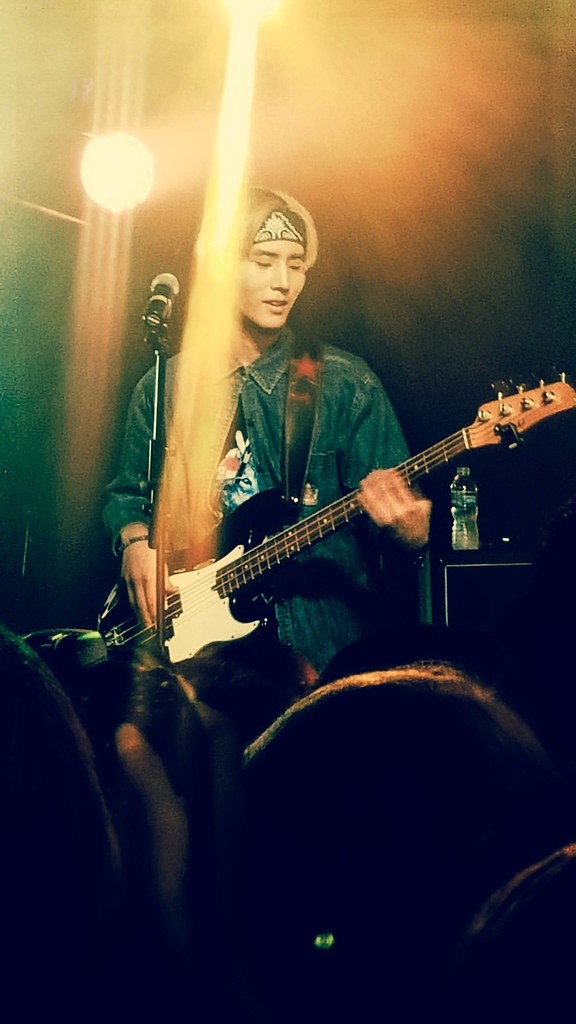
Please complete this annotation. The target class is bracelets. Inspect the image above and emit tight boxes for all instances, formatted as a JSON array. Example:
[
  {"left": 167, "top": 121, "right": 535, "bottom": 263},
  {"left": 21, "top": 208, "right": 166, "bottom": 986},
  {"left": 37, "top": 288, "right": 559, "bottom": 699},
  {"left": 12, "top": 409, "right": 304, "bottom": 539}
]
[{"left": 119, "top": 534, "right": 149, "bottom": 551}]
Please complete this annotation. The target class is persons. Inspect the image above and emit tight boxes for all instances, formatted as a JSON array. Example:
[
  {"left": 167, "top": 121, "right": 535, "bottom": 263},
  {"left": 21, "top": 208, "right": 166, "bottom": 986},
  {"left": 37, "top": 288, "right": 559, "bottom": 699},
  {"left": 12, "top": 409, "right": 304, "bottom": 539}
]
[
  {"left": 101, "top": 185, "right": 430, "bottom": 791},
  {"left": 1, "top": 624, "right": 576, "bottom": 1023}
]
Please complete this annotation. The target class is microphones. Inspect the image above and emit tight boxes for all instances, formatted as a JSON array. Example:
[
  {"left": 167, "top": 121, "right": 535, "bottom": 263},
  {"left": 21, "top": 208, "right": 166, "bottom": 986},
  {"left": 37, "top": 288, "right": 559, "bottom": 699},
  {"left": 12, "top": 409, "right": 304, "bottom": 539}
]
[{"left": 142, "top": 273, "right": 180, "bottom": 333}]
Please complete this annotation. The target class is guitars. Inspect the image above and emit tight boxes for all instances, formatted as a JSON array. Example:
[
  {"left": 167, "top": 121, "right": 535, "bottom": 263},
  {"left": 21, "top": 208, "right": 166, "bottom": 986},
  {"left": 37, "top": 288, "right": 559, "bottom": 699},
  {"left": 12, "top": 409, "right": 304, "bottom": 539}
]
[{"left": 97, "top": 362, "right": 576, "bottom": 682}]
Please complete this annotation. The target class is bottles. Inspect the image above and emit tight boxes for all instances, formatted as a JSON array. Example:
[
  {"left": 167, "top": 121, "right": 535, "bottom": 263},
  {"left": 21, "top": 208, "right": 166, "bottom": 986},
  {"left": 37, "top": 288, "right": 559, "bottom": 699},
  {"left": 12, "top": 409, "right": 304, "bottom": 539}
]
[{"left": 450, "top": 467, "right": 479, "bottom": 550}]
